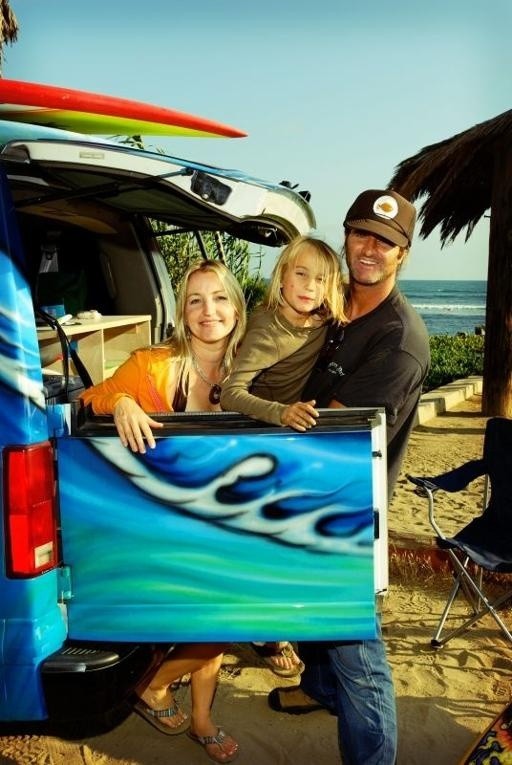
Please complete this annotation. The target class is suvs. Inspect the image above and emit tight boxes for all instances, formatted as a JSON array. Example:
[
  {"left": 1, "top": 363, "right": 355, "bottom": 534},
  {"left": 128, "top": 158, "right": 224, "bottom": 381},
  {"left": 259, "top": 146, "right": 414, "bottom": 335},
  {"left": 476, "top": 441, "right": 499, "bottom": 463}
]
[{"left": 0, "top": 75, "right": 395, "bottom": 739}]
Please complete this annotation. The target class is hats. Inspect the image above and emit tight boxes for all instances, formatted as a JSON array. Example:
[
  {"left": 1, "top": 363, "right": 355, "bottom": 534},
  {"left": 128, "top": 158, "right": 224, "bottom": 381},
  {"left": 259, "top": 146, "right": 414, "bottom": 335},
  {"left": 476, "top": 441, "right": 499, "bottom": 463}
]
[{"left": 343, "top": 188, "right": 417, "bottom": 245}]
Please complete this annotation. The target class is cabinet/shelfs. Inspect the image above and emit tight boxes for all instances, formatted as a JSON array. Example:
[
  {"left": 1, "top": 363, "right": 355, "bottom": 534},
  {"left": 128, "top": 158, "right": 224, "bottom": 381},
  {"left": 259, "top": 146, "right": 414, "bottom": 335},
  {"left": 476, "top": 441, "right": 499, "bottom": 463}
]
[{"left": 36, "top": 311, "right": 153, "bottom": 391}]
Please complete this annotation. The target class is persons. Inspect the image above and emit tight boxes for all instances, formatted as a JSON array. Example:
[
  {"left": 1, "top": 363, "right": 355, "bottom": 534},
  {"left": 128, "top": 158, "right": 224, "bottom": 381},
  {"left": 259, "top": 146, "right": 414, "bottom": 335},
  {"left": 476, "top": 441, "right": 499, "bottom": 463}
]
[
  {"left": 219, "top": 234, "right": 356, "bottom": 680},
  {"left": 264, "top": 189, "right": 433, "bottom": 765},
  {"left": 77, "top": 261, "right": 250, "bottom": 763}
]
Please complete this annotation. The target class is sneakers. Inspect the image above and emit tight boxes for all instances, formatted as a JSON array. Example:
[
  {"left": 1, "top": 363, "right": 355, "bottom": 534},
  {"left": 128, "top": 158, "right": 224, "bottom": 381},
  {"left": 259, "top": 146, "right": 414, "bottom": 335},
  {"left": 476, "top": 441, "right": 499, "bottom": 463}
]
[{"left": 268, "top": 686, "right": 328, "bottom": 715}]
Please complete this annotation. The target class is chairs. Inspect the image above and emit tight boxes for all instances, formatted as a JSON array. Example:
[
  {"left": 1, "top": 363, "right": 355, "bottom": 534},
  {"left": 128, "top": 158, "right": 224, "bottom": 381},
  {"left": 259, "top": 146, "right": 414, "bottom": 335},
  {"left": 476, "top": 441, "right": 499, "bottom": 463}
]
[{"left": 404, "top": 417, "right": 512, "bottom": 653}]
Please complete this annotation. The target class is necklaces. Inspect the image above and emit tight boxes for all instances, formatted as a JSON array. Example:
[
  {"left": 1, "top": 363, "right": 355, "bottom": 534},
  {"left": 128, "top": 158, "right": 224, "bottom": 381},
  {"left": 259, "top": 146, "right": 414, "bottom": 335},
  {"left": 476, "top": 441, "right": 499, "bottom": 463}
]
[{"left": 188, "top": 339, "right": 227, "bottom": 405}]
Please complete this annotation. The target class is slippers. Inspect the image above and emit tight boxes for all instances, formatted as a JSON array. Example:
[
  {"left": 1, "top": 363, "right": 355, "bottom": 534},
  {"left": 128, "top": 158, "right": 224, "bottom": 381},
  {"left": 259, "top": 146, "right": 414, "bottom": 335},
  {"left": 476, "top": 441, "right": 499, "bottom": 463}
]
[
  {"left": 251, "top": 641, "right": 306, "bottom": 678},
  {"left": 187, "top": 725, "right": 239, "bottom": 763},
  {"left": 134, "top": 699, "right": 191, "bottom": 736}
]
